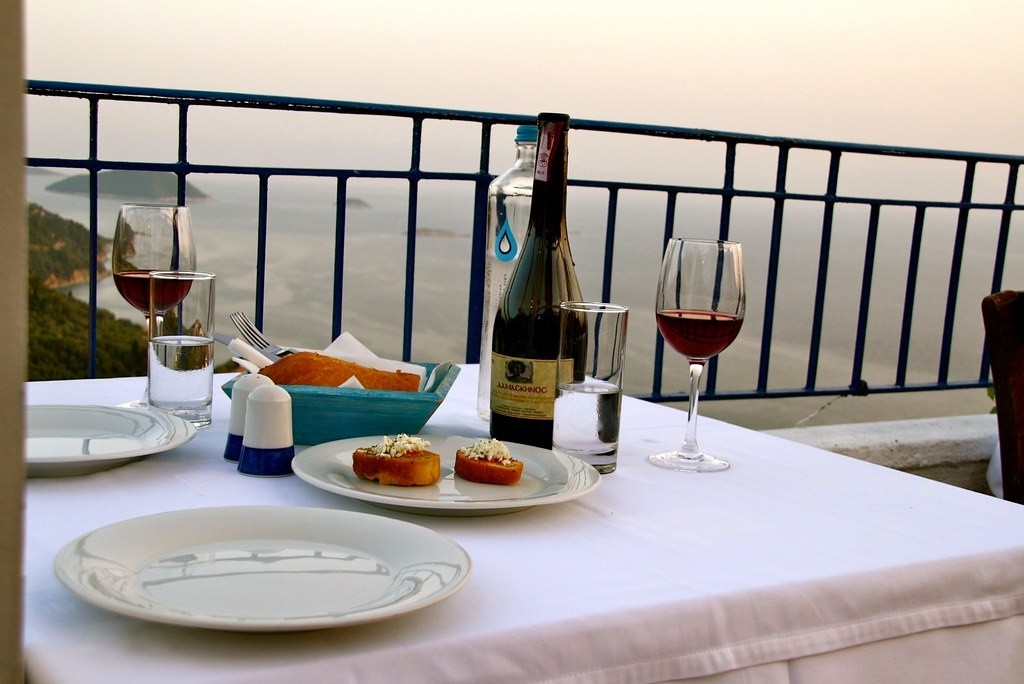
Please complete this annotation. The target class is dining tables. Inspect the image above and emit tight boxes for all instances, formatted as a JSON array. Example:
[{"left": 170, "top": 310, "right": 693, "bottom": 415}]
[{"left": 24, "top": 362, "right": 1024, "bottom": 684}]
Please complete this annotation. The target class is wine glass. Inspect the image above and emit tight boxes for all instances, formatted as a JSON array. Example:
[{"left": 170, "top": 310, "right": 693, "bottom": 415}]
[
  {"left": 112, "top": 204, "right": 198, "bottom": 421},
  {"left": 646, "top": 237, "right": 746, "bottom": 472}
]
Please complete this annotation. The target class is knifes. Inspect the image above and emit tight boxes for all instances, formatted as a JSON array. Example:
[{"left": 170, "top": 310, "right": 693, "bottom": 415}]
[{"left": 207, "top": 332, "right": 282, "bottom": 365}]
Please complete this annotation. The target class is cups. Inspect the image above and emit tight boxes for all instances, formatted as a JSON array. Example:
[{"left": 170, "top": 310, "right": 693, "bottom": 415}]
[
  {"left": 146, "top": 269, "right": 217, "bottom": 430},
  {"left": 551, "top": 301, "right": 627, "bottom": 475}
]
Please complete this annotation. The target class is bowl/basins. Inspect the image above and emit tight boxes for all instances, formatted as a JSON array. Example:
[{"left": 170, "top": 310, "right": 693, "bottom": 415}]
[{"left": 221, "top": 360, "right": 463, "bottom": 444}]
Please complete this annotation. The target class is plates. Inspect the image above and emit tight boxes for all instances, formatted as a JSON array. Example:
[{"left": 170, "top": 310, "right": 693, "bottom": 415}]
[
  {"left": 291, "top": 434, "right": 602, "bottom": 519},
  {"left": 24, "top": 404, "right": 198, "bottom": 477},
  {"left": 54, "top": 504, "right": 472, "bottom": 632}
]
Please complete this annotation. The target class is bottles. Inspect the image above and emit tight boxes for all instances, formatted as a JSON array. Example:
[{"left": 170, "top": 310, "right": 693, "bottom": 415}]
[
  {"left": 476, "top": 124, "right": 541, "bottom": 420},
  {"left": 493, "top": 111, "right": 589, "bottom": 452}
]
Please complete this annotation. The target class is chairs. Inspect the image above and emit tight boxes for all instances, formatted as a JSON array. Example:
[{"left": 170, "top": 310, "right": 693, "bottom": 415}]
[{"left": 980, "top": 289, "right": 1024, "bottom": 506}]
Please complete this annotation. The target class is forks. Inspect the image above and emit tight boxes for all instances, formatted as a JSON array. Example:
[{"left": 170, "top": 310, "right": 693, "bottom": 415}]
[{"left": 230, "top": 312, "right": 305, "bottom": 358}]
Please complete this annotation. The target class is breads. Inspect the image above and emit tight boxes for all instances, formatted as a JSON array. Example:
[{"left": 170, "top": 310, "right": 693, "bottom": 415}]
[
  {"left": 455, "top": 449, "right": 524, "bottom": 485},
  {"left": 353, "top": 445, "right": 440, "bottom": 485},
  {"left": 256, "top": 351, "right": 421, "bottom": 394}
]
